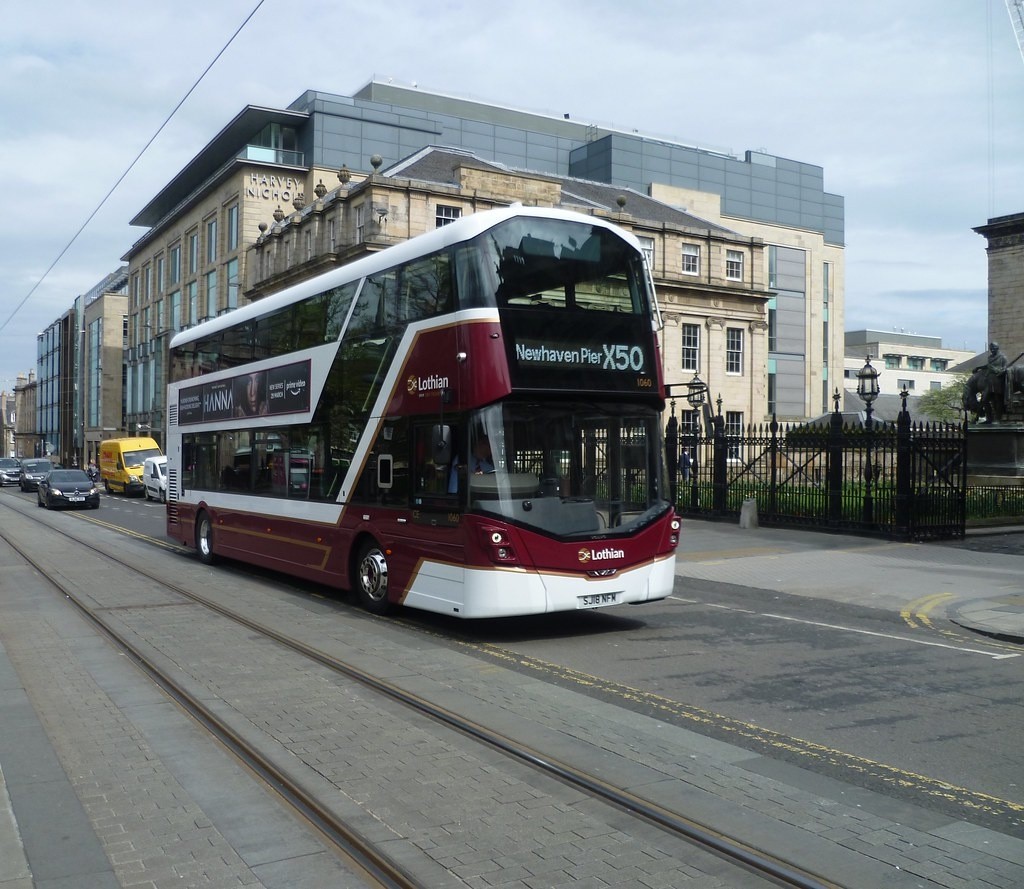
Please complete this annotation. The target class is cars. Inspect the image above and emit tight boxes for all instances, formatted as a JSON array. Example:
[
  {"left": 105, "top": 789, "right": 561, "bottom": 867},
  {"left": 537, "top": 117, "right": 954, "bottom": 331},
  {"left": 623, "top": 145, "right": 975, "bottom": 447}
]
[
  {"left": 19, "top": 464, "right": 53, "bottom": 492},
  {"left": 0, "top": 458, "right": 24, "bottom": 487},
  {"left": 37, "top": 469, "right": 100, "bottom": 511}
]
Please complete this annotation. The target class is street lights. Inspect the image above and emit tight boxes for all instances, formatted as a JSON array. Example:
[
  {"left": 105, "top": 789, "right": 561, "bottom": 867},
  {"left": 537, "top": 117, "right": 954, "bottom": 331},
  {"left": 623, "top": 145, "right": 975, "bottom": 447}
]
[
  {"left": 686, "top": 369, "right": 706, "bottom": 509},
  {"left": 854, "top": 354, "right": 882, "bottom": 524}
]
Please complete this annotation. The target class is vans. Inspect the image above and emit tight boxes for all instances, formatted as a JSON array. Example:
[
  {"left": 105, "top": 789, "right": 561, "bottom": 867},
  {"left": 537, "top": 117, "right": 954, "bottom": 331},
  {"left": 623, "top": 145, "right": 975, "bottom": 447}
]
[
  {"left": 98, "top": 437, "right": 164, "bottom": 499},
  {"left": 143, "top": 456, "right": 168, "bottom": 504}
]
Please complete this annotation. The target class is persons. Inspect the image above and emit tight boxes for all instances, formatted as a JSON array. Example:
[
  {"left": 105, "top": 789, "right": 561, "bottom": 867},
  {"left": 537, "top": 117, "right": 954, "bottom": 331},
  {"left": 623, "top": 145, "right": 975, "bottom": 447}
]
[
  {"left": 234, "top": 371, "right": 267, "bottom": 418},
  {"left": 449, "top": 437, "right": 500, "bottom": 493}
]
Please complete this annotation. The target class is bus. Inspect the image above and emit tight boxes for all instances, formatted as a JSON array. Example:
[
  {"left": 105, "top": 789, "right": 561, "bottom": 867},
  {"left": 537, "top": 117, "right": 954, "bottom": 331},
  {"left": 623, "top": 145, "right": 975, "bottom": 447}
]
[{"left": 167, "top": 200, "right": 681, "bottom": 619}]
